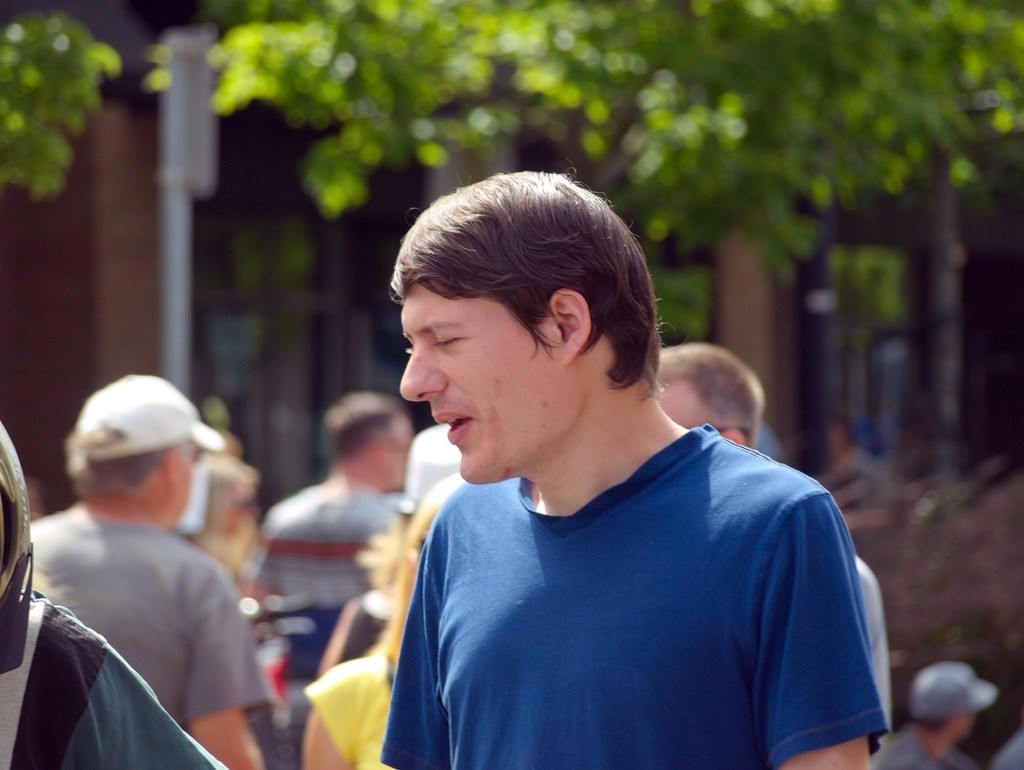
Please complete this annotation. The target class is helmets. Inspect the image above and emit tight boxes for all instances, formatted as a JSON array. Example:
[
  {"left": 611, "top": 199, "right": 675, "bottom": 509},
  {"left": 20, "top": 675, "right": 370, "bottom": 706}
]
[{"left": 0, "top": 423, "right": 34, "bottom": 675}]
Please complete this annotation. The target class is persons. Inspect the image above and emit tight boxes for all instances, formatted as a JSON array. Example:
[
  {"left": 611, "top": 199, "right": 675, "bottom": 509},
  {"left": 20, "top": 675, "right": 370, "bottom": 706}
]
[
  {"left": 379, "top": 171, "right": 890, "bottom": 770},
  {"left": 873, "top": 660, "right": 999, "bottom": 770},
  {"left": 0, "top": 375, "right": 460, "bottom": 770},
  {"left": 654, "top": 341, "right": 891, "bottom": 731}
]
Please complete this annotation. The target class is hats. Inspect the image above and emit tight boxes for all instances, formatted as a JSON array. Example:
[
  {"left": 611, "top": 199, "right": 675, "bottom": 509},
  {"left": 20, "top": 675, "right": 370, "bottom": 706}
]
[
  {"left": 63, "top": 373, "right": 229, "bottom": 464},
  {"left": 400, "top": 422, "right": 459, "bottom": 515},
  {"left": 907, "top": 660, "right": 1002, "bottom": 723}
]
